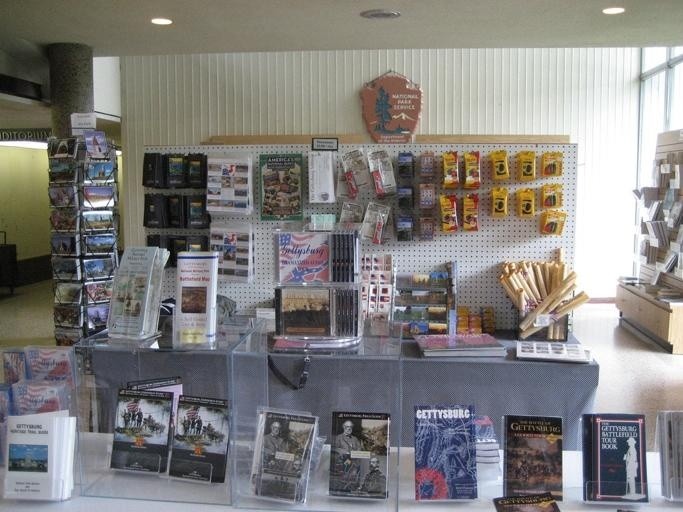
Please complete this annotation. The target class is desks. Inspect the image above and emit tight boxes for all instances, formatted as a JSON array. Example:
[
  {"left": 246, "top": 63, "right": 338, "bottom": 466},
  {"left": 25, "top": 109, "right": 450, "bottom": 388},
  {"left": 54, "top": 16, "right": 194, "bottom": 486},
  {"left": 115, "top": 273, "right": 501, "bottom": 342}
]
[
  {"left": 74, "top": 329, "right": 599, "bottom": 452},
  {"left": 0, "top": 320, "right": 683, "bottom": 511},
  {"left": 0, "top": 244, "right": 19, "bottom": 294}
]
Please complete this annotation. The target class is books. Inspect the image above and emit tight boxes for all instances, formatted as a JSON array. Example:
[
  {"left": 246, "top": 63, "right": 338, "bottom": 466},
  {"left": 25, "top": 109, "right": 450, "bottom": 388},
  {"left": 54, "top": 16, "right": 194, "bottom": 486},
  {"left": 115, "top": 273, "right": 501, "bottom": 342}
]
[
  {"left": 582, "top": 411, "right": 683, "bottom": 502},
  {"left": 414, "top": 334, "right": 505, "bottom": 357},
  {"left": 0, "top": 345, "right": 99, "bottom": 502},
  {"left": 251, "top": 411, "right": 390, "bottom": 503},
  {"left": 618, "top": 151, "right": 683, "bottom": 302},
  {"left": 111, "top": 377, "right": 234, "bottom": 482},
  {"left": 413, "top": 405, "right": 563, "bottom": 512}
]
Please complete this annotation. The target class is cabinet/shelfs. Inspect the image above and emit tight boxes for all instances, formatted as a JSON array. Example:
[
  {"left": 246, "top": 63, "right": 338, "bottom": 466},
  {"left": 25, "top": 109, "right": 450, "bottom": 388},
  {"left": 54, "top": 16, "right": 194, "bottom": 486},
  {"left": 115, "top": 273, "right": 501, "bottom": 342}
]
[
  {"left": 617, "top": 130, "right": 683, "bottom": 356},
  {"left": 45, "top": 127, "right": 120, "bottom": 345}
]
